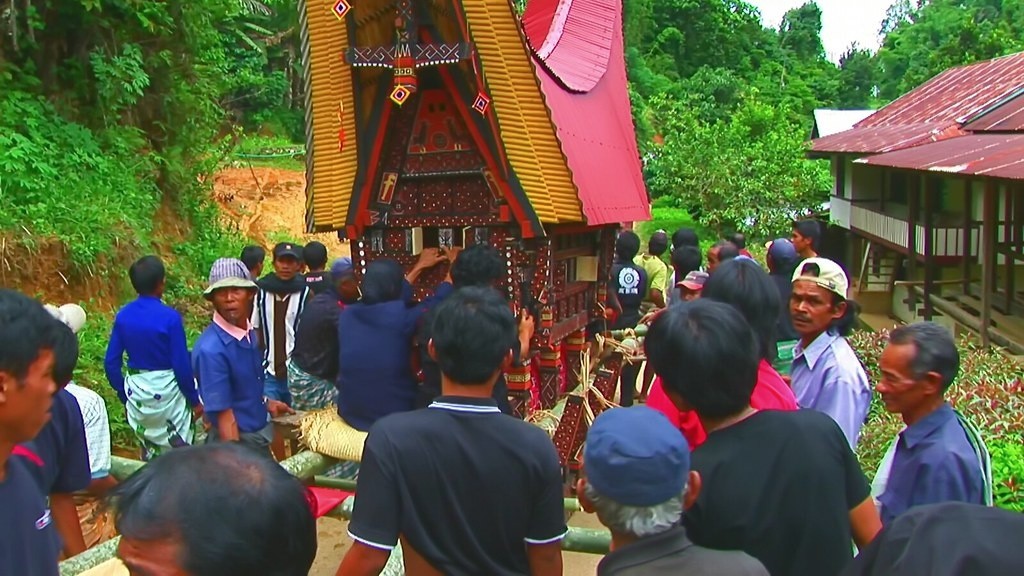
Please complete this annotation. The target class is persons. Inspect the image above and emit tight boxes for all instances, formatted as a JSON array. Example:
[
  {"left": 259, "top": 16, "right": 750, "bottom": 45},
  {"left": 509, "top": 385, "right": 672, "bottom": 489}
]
[{"left": 0, "top": 218, "right": 1024, "bottom": 576}]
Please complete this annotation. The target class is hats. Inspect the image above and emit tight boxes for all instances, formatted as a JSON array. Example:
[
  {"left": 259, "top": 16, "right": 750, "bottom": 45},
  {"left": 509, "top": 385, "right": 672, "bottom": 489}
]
[
  {"left": 791, "top": 257, "right": 850, "bottom": 301},
  {"left": 584, "top": 405, "right": 691, "bottom": 507},
  {"left": 274, "top": 242, "right": 300, "bottom": 262},
  {"left": 203, "top": 257, "right": 260, "bottom": 301},
  {"left": 332, "top": 257, "right": 352, "bottom": 275},
  {"left": 43, "top": 303, "right": 87, "bottom": 334},
  {"left": 676, "top": 270, "right": 710, "bottom": 291}
]
